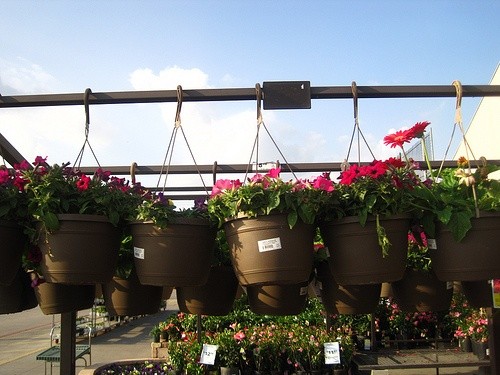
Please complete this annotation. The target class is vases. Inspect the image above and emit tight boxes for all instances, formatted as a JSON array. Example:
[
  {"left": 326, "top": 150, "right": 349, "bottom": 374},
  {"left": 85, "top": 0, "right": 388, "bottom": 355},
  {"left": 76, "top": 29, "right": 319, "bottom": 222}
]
[
  {"left": 392, "top": 270, "right": 455, "bottom": 317},
  {"left": 34, "top": 282, "right": 97, "bottom": 315},
  {"left": 33, "top": 214, "right": 121, "bottom": 283},
  {"left": 246, "top": 285, "right": 308, "bottom": 316},
  {"left": 431, "top": 211, "right": 500, "bottom": 283},
  {"left": 222, "top": 211, "right": 314, "bottom": 287},
  {"left": 177, "top": 268, "right": 239, "bottom": 316},
  {"left": 319, "top": 214, "right": 410, "bottom": 287},
  {"left": 462, "top": 280, "right": 493, "bottom": 309},
  {"left": 127, "top": 218, "right": 218, "bottom": 288},
  {"left": 321, "top": 285, "right": 382, "bottom": 315},
  {"left": 101, "top": 274, "right": 161, "bottom": 315}
]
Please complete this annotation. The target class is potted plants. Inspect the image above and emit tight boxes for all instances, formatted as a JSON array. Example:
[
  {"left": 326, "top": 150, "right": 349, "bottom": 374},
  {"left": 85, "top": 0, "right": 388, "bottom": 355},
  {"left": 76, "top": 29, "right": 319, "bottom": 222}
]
[{"left": 53, "top": 306, "right": 122, "bottom": 343}]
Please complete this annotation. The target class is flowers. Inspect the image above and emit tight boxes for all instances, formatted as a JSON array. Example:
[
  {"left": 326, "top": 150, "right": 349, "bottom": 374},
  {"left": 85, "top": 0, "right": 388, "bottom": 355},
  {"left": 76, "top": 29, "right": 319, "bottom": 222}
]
[
  {"left": 0, "top": 121, "right": 500, "bottom": 272},
  {"left": 150, "top": 305, "right": 490, "bottom": 375}
]
